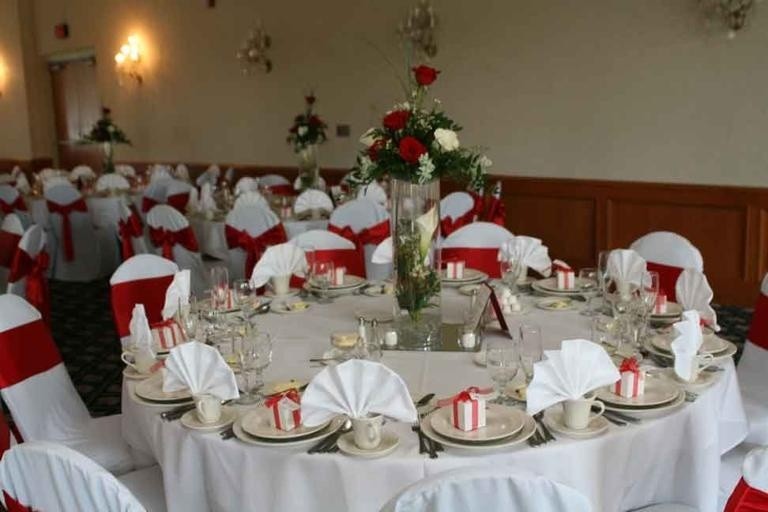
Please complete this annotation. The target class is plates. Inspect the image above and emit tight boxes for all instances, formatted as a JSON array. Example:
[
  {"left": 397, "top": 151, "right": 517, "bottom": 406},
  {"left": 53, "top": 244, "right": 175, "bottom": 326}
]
[
  {"left": 604, "top": 388, "right": 684, "bottom": 418},
  {"left": 233, "top": 410, "right": 345, "bottom": 449},
  {"left": 419, "top": 411, "right": 537, "bottom": 447},
  {"left": 435, "top": 267, "right": 489, "bottom": 287},
  {"left": 431, "top": 404, "right": 524, "bottom": 440},
  {"left": 596, "top": 379, "right": 678, "bottom": 404},
  {"left": 529, "top": 276, "right": 739, "bottom": 361},
  {"left": 134, "top": 375, "right": 190, "bottom": 409},
  {"left": 545, "top": 412, "right": 607, "bottom": 439},
  {"left": 305, "top": 273, "right": 366, "bottom": 296},
  {"left": 242, "top": 407, "right": 329, "bottom": 439},
  {"left": 180, "top": 409, "right": 237, "bottom": 431},
  {"left": 335, "top": 431, "right": 400, "bottom": 448}
]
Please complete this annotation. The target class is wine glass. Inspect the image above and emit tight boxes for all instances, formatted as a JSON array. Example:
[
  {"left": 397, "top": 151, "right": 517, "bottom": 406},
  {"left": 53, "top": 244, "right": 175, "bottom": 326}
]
[
  {"left": 481, "top": 324, "right": 540, "bottom": 404},
  {"left": 571, "top": 258, "right": 660, "bottom": 364},
  {"left": 180, "top": 260, "right": 271, "bottom": 409},
  {"left": 296, "top": 242, "right": 335, "bottom": 310}
]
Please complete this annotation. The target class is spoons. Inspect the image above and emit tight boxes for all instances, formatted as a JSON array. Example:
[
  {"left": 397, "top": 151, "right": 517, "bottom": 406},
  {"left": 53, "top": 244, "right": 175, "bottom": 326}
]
[
  {"left": 247, "top": 306, "right": 271, "bottom": 319},
  {"left": 415, "top": 393, "right": 435, "bottom": 409}
]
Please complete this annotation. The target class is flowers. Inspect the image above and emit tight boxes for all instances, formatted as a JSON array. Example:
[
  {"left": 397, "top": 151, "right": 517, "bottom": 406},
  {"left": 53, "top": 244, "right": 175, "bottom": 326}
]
[
  {"left": 352, "top": 67, "right": 505, "bottom": 194},
  {"left": 281, "top": 92, "right": 332, "bottom": 146},
  {"left": 75, "top": 109, "right": 137, "bottom": 143}
]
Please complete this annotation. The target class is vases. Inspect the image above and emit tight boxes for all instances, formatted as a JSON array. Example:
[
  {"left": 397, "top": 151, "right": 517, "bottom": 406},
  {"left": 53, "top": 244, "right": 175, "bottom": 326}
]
[
  {"left": 95, "top": 139, "right": 115, "bottom": 171},
  {"left": 299, "top": 146, "right": 324, "bottom": 193}
]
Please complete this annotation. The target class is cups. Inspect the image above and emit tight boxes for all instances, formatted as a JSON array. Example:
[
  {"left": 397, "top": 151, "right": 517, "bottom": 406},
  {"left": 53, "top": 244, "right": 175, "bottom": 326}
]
[
  {"left": 346, "top": 408, "right": 385, "bottom": 448},
  {"left": 186, "top": 388, "right": 220, "bottom": 421},
  {"left": 675, "top": 351, "right": 712, "bottom": 383},
  {"left": 120, "top": 344, "right": 161, "bottom": 376},
  {"left": 558, "top": 387, "right": 606, "bottom": 429}
]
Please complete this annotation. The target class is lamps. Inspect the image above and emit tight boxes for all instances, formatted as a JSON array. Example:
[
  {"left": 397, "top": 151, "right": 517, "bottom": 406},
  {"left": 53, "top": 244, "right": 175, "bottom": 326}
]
[
  {"left": 109, "top": 30, "right": 146, "bottom": 83},
  {"left": 403, "top": 2, "right": 441, "bottom": 56},
  {"left": 234, "top": 21, "right": 275, "bottom": 74},
  {"left": 700, "top": 0, "right": 752, "bottom": 37}
]
